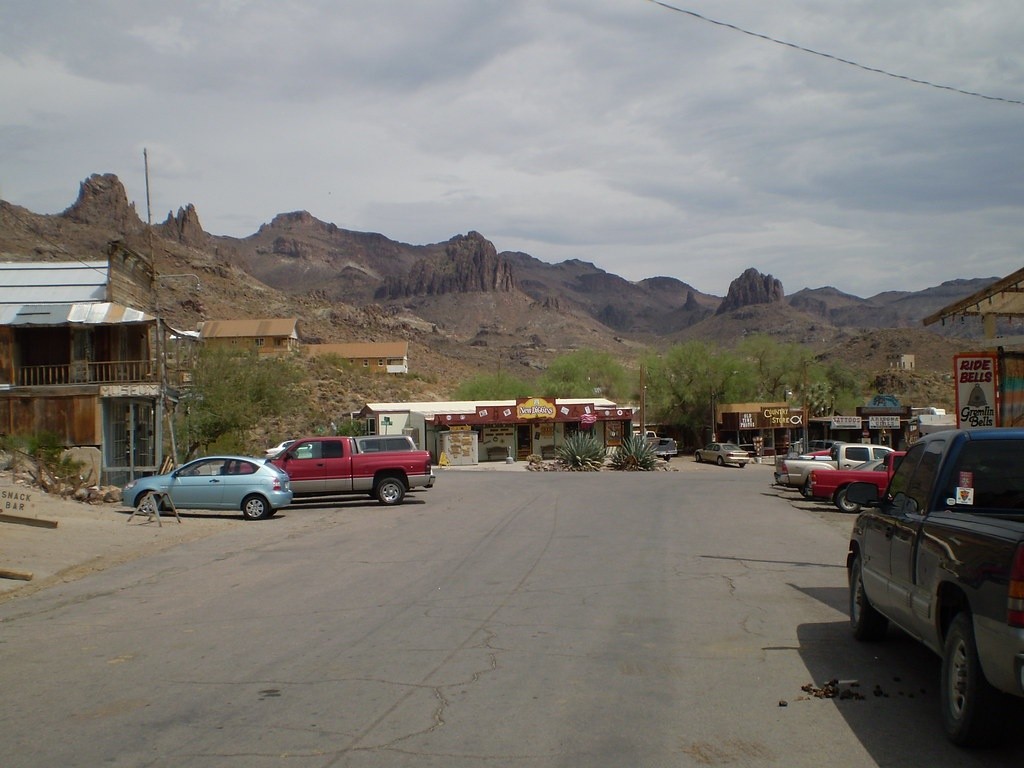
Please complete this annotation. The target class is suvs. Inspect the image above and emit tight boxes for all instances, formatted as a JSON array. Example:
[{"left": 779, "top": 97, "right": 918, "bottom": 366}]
[
  {"left": 809, "top": 440, "right": 846, "bottom": 450},
  {"left": 266, "top": 436, "right": 416, "bottom": 458}
]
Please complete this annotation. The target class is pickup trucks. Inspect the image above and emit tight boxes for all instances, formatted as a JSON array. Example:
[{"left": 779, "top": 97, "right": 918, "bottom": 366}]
[
  {"left": 846, "top": 426, "right": 1024, "bottom": 746},
  {"left": 220, "top": 436, "right": 436, "bottom": 506},
  {"left": 633, "top": 429, "right": 678, "bottom": 462},
  {"left": 774, "top": 438, "right": 895, "bottom": 501},
  {"left": 807, "top": 450, "right": 909, "bottom": 514}
]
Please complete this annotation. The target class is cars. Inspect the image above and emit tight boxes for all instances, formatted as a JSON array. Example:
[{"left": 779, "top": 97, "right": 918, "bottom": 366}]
[
  {"left": 694, "top": 442, "right": 750, "bottom": 468},
  {"left": 119, "top": 456, "right": 293, "bottom": 520}
]
